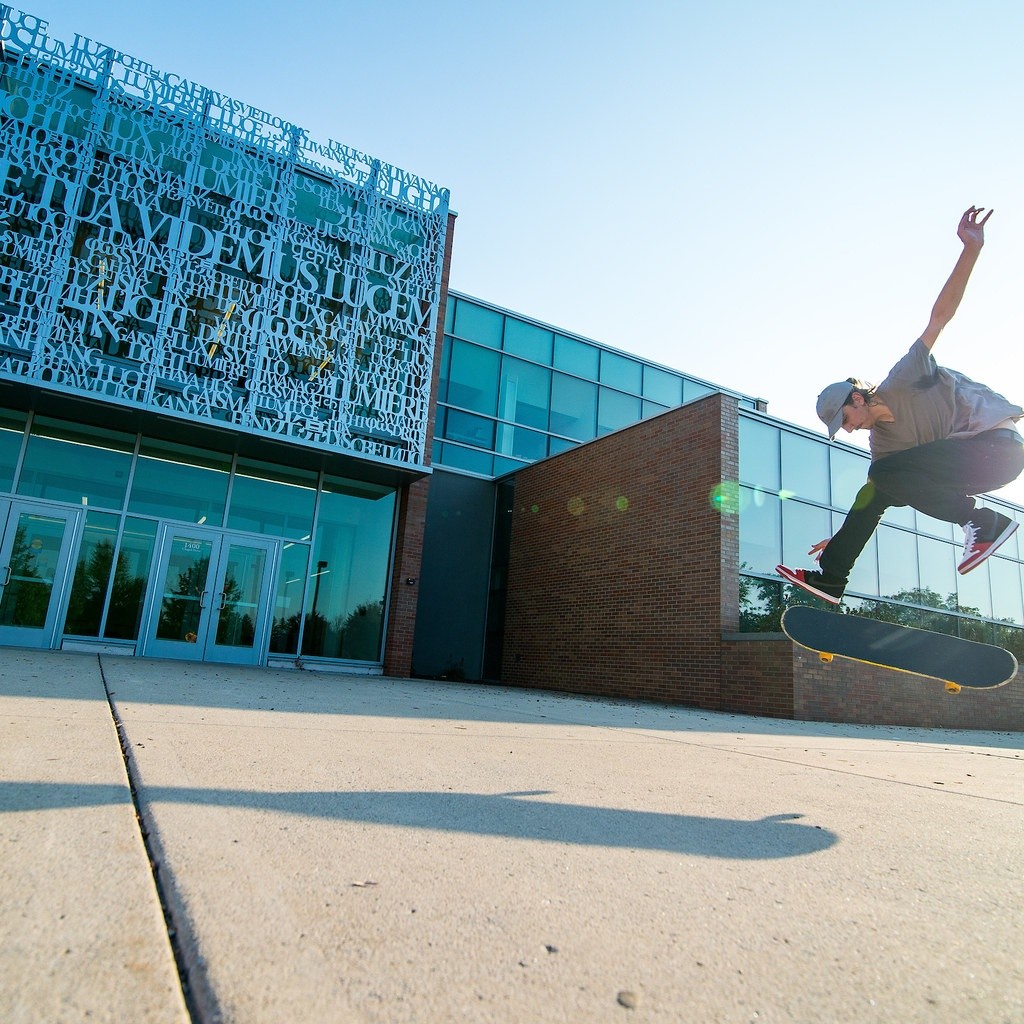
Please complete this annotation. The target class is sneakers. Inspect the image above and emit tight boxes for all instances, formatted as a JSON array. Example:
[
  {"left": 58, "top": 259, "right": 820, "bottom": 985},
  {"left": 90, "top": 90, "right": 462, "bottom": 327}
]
[
  {"left": 958, "top": 507, "right": 1020, "bottom": 575},
  {"left": 775, "top": 564, "right": 849, "bottom": 605}
]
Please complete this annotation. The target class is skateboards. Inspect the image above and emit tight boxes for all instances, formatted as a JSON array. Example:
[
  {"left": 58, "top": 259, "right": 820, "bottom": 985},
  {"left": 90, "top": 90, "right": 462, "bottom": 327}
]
[{"left": 780, "top": 605, "right": 1018, "bottom": 694}]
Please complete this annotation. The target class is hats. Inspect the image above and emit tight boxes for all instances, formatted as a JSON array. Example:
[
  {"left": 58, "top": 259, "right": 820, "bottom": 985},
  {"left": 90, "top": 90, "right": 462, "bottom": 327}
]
[{"left": 816, "top": 381, "right": 854, "bottom": 443}]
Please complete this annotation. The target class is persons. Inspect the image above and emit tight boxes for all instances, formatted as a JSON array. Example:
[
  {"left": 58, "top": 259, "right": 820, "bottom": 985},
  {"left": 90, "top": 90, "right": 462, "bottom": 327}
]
[{"left": 775, "top": 202, "right": 1023, "bottom": 606}]
